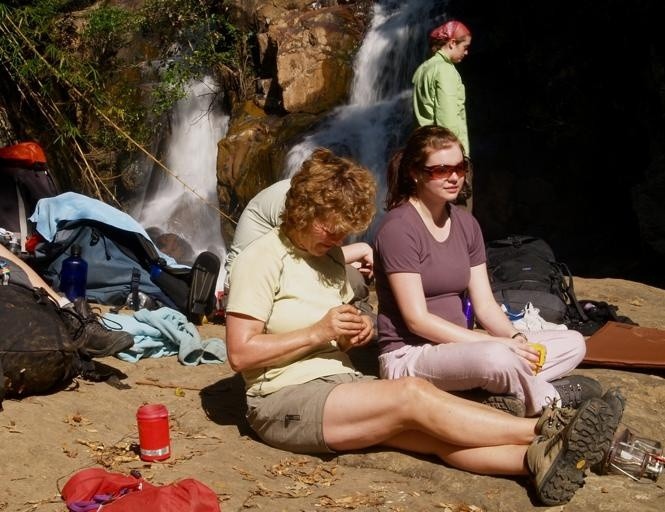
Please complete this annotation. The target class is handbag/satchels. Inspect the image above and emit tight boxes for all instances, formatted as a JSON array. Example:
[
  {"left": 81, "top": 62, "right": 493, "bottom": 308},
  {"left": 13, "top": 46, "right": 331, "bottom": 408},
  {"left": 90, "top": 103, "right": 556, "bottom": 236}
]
[{"left": 0, "top": 277, "right": 81, "bottom": 400}]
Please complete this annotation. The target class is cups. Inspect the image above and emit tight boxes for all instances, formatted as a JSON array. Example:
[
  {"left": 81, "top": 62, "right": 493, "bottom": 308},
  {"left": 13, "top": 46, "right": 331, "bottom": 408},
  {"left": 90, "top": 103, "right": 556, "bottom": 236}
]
[
  {"left": 527, "top": 342, "right": 547, "bottom": 376},
  {"left": 135, "top": 403, "right": 171, "bottom": 461}
]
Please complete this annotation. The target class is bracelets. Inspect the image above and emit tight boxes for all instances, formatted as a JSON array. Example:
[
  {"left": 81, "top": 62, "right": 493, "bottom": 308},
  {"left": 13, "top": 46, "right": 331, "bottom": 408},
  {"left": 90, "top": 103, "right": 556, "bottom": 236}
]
[{"left": 512, "top": 332, "right": 527, "bottom": 340}]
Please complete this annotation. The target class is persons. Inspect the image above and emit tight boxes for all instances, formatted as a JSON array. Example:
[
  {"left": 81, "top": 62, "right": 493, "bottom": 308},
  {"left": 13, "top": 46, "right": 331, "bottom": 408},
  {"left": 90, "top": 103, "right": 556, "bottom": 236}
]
[
  {"left": 224, "top": 148, "right": 624, "bottom": 506},
  {"left": 0, "top": 244, "right": 134, "bottom": 358},
  {"left": 373, "top": 125, "right": 603, "bottom": 419},
  {"left": 223, "top": 142, "right": 374, "bottom": 305},
  {"left": 412, "top": 21, "right": 474, "bottom": 213}
]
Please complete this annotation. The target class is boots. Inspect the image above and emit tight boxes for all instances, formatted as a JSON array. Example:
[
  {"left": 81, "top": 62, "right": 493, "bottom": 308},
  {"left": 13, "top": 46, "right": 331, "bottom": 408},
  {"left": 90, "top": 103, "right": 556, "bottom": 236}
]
[{"left": 62, "top": 298, "right": 133, "bottom": 357}]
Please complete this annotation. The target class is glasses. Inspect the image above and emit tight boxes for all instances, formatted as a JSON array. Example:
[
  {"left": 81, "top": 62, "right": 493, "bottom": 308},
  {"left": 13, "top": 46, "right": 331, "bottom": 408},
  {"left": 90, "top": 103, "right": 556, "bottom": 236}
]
[{"left": 420, "top": 156, "right": 470, "bottom": 181}]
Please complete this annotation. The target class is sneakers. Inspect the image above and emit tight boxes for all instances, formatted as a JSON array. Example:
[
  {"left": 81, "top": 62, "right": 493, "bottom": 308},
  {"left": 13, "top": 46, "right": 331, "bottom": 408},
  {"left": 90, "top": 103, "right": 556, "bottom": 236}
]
[{"left": 481, "top": 373, "right": 628, "bottom": 506}]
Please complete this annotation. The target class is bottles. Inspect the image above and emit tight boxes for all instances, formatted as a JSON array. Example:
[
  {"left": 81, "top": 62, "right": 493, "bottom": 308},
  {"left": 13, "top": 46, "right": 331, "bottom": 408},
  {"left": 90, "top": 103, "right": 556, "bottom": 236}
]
[
  {"left": 148, "top": 265, "right": 163, "bottom": 280},
  {"left": 59, "top": 243, "right": 88, "bottom": 301},
  {"left": 462, "top": 291, "right": 476, "bottom": 331},
  {"left": 609, "top": 427, "right": 665, "bottom": 483}
]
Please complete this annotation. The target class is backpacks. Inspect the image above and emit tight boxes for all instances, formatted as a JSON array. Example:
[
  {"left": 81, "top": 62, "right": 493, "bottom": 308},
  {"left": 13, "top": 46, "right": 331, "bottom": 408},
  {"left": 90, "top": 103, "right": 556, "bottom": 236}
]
[{"left": 484, "top": 233, "right": 571, "bottom": 322}]
